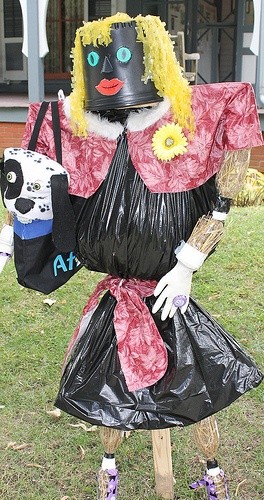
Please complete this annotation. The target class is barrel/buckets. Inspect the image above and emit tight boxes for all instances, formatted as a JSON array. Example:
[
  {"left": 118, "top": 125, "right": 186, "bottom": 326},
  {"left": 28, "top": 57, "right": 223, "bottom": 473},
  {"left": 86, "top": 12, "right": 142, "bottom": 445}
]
[{"left": 80, "top": 21, "right": 163, "bottom": 111}]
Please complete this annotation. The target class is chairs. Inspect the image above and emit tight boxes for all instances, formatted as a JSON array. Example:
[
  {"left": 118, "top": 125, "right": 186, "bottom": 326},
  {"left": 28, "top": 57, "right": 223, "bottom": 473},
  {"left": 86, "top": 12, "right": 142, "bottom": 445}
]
[{"left": 170, "top": 31, "right": 201, "bottom": 84}]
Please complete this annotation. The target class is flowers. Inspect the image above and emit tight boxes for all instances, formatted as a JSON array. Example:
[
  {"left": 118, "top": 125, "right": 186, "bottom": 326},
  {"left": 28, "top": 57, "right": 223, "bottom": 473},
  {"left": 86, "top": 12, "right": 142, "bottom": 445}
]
[{"left": 152, "top": 122, "right": 190, "bottom": 163}]
